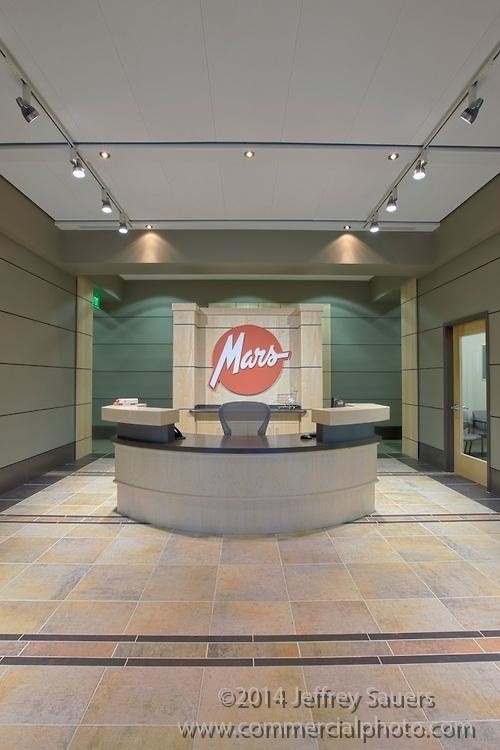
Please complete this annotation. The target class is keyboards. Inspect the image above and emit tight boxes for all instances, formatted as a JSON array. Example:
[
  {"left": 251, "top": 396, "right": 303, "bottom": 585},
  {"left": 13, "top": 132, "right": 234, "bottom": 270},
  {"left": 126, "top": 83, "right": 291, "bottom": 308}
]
[{"left": 309, "top": 433, "right": 316, "bottom": 437}]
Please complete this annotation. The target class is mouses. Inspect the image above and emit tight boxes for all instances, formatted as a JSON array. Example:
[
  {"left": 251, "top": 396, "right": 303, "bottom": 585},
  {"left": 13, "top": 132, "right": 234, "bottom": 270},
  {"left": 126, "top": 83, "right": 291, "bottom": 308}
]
[{"left": 301, "top": 435, "right": 312, "bottom": 439}]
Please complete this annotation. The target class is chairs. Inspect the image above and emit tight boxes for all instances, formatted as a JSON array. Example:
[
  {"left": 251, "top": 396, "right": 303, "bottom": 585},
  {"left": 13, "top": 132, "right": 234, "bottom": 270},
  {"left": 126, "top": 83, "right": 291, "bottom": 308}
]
[
  {"left": 464, "top": 410, "right": 488, "bottom": 458},
  {"left": 220, "top": 401, "right": 271, "bottom": 436}
]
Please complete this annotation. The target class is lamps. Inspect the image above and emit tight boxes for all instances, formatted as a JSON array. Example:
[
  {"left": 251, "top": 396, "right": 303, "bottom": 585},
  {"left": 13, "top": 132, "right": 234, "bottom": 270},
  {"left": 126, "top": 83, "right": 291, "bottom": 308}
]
[
  {"left": 17, "top": 83, "right": 130, "bottom": 235},
  {"left": 362, "top": 83, "right": 484, "bottom": 233}
]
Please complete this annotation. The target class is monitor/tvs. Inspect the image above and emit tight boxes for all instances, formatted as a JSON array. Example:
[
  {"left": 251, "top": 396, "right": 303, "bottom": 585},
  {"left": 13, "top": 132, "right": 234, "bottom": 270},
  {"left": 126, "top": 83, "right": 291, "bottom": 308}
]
[{"left": 331, "top": 398, "right": 345, "bottom": 407}]
